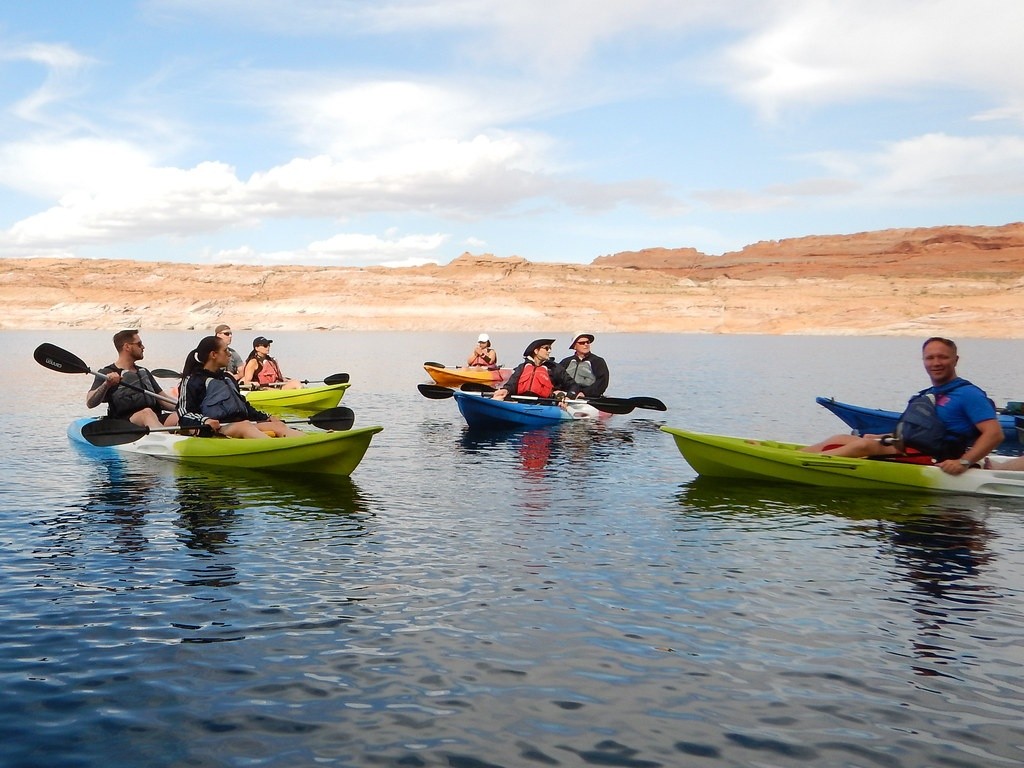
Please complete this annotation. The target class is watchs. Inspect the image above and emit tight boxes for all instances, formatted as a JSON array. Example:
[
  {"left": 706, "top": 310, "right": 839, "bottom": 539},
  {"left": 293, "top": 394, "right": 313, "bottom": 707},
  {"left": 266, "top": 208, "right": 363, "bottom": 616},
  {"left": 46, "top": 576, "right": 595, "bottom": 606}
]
[{"left": 958, "top": 457, "right": 970, "bottom": 468}]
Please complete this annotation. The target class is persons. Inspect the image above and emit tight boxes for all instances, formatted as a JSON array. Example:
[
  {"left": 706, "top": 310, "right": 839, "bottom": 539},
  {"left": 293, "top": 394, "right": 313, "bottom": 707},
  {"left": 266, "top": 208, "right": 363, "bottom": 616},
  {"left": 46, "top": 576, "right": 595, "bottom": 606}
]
[
  {"left": 243, "top": 337, "right": 301, "bottom": 390},
  {"left": 559, "top": 332, "right": 609, "bottom": 399},
  {"left": 489, "top": 340, "right": 579, "bottom": 409},
  {"left": 466, "top": 334, "right": 497, "bottom": 371},
  {"left": 215, "top": 325, "right": 245, "bottom": 382},
  {"left": 175, "top": 336, "right": 311, "bottom": 440},
  {"left": 798, "top": 336, "right": 1005, "bottom": 475},
  {"left": 85, "top": 329, "right": 179, "bottom": 435}
]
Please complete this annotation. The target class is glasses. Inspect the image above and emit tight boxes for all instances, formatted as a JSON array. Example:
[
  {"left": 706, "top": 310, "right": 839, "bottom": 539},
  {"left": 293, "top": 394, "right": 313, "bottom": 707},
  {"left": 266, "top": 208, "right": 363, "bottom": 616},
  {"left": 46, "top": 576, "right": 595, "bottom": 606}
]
[
  {"left": 576, "top": 340, "right": 590, "bottom": 345},
  {"left": 214, "top": 348, "right": 229, "bottom": 355},
  {"left": 258, "top": 343, "right": 270, "bottom": 348},
  {"left": 539, "top": 346, "right": 551, "bottom": 351},
  {"left": 220, "top": 331, "right": 233, "bottom": 336},
  {"left": 122, "top": 341, "right": 142, "bottom": 348}
]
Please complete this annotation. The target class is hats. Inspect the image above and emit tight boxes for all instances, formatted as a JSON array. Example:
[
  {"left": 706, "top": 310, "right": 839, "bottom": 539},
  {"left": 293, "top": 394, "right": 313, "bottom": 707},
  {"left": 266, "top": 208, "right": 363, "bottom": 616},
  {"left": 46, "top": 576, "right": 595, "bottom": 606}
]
[
  {"left": 254, "top": 337, "right": 274, "bottom": 346},
  {"left": 215, "top": 325, "right": 230, "bottom": 337},
  {"left": 569, "top": 332, "right": 595, "bottom": 349},
  {"left": 524, "top": 338, "right": 556, "bottom": 356},
  {"left": 477, "top": 334, "right": 491, "bottom": 347}
]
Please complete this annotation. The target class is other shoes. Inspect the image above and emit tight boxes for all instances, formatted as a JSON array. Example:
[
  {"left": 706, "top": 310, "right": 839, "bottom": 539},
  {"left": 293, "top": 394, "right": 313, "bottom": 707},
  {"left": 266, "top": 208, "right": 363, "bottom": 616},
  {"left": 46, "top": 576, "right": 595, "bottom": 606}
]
[{"left": 557, "top": 392, "right": 565, "bottom": 399}]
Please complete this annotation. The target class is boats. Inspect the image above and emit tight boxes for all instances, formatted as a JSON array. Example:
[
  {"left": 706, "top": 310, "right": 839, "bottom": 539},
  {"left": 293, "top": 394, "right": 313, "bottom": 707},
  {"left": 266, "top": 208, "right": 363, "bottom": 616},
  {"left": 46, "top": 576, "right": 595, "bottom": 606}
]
[
  {"left": 170, "top": 383, "right": 352, "bottom": 417},
  {"left": 425, "top": 365, "right": 516, "bottom": 387},
  {"left": 67, "top": 416, "right": 384, "bottom": 477},
  {"left": 816, "top": 396, "right": 1024, "bottom": 444},
  {"left": 660, "top": 426, "right": 1024, "bottom": 498},
  {"left": 454, "top": 391, "right": 613, "bottom": 431}
]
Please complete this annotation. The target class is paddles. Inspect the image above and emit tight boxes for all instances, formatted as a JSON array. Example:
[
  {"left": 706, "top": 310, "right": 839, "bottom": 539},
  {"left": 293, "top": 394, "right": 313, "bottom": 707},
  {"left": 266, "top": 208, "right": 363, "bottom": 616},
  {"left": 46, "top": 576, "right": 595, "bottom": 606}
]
[
  {"left": 417, "top": 384, "right": 638, "bottom": 414},
  {"left": 81, "top": 406, "right": 355, "bottom": 447},
  {"left": 239, "top": 373, "right": 350, "bottom": 386},
  {"left": 151, "top": 369, "right": 184, "bottom": 379},
  {"left": 459, "top": 382, "right": 668, "bottom": 411},
  {"left": 424, "top": 362, "right": 517, "bottom": 371},
  {"left": 33, "top": 343, "right": 178, "bottom": 405},
  {"left": 874, "top": 435, "right": 900, "bottom": 446}
]
